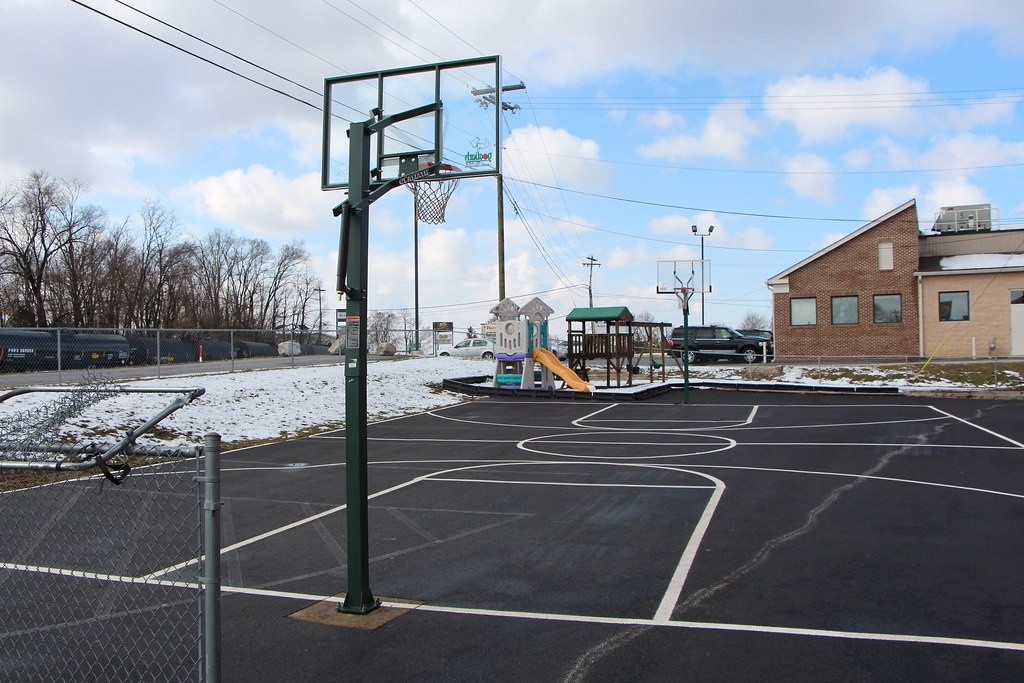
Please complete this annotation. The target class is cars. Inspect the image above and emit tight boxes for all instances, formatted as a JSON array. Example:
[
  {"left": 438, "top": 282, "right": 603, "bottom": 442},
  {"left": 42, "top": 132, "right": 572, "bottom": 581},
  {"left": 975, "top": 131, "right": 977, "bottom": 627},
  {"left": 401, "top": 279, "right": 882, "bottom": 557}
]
[
  {"left": 552, "top": 339, "right": 582, "bottom": 356},
  {"left": 436, "top": 337, "right": 497, "bottom": 361}
]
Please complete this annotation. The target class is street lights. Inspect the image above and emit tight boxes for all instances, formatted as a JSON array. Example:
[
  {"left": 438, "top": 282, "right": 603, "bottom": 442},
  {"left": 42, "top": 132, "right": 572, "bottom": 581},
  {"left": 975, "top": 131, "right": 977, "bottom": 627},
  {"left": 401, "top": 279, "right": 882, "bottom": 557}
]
[{"left": 692, "top": 225, "right": 714, "bottom": 324}]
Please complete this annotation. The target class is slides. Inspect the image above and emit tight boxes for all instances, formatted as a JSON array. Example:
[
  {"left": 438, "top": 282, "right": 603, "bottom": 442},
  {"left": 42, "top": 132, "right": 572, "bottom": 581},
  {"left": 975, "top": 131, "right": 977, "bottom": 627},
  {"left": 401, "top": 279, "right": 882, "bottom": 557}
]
[{"left": 532, "top": 347, "right": 595, "bottom": 393}]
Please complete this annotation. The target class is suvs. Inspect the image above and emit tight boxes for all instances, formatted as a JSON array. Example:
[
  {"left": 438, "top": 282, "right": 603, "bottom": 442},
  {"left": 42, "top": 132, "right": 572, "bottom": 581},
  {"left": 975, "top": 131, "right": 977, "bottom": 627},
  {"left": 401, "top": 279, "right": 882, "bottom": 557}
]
[
  {"left": 725, "top": 328, "right": 773, "bottom": 362},
  {"left": 666, "top": 324, "right": 775, "bottom": 366}
]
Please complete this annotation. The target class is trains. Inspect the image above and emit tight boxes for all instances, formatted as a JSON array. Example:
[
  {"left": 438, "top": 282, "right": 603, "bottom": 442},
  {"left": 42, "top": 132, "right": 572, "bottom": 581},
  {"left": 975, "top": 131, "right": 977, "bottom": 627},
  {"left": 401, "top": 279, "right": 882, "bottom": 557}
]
[{"left": 0, "top": 331, "right": 337, "bottom": 375}]
